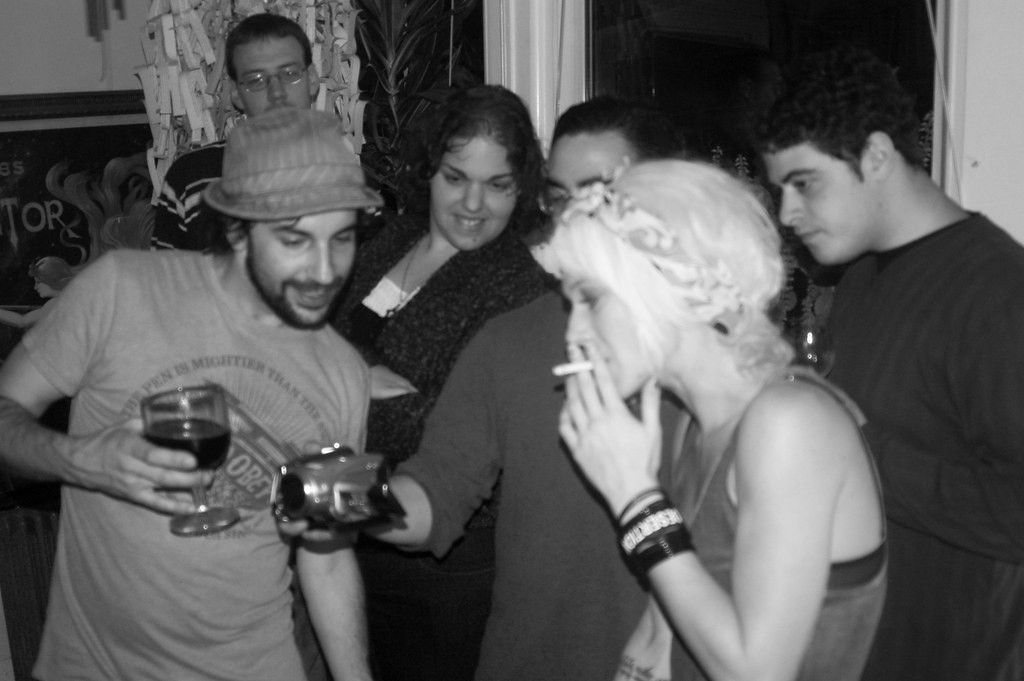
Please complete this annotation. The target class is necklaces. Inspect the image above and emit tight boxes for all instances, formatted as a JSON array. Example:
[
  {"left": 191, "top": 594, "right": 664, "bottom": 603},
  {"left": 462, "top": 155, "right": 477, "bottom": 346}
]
[{"left": 388, "top": 235, "right": 420, "bottom": 317}]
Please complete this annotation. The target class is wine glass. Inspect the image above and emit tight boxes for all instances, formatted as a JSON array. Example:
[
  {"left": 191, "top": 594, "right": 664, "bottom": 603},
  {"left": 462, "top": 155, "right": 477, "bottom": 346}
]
[{"left": 140, "top": 385, "right": 239, "bottom": 534}]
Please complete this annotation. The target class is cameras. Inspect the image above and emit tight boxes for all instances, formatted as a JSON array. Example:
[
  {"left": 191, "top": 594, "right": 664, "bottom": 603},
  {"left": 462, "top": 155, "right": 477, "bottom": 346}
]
[{"left": 272, "top": 445, "right": 408, "bottom": 527}]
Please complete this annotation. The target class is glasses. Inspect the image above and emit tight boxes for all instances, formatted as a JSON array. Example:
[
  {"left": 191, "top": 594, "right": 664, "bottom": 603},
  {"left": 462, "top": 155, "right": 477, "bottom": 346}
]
[
  {"left": 237, "top": 64, "right": 311, "bottom": 93},
  {"left": 537, "top": 182, "right": 574, "bottom": 216}
]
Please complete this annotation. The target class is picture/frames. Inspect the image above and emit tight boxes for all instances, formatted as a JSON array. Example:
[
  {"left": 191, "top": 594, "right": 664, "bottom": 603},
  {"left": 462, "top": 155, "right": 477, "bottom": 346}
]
[{"left": 0, "top": 89, "right": 153, "bottom": 510}]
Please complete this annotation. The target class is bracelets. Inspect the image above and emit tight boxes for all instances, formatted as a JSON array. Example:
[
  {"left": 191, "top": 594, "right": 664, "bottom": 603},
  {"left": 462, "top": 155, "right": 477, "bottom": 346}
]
[{"left": 615, "top": 488, "right": 695, "bottom": 573}]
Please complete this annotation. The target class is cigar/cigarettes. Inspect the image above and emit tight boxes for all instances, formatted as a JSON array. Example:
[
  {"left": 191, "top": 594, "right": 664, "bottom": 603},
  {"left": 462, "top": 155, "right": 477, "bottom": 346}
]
[{"left": 548, "top": 359, "right": 596, "bottom": 377}]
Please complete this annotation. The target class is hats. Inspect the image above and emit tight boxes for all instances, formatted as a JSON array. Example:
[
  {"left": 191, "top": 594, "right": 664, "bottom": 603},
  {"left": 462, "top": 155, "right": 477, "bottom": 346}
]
[{"left": 201, "top": 108, "right": 383, "bottom": 220}]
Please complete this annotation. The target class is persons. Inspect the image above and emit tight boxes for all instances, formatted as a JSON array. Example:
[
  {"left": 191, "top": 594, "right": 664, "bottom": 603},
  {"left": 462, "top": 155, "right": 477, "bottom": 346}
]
[{"left": 0, "top": 15, "right": 1024, "bottom": 681}]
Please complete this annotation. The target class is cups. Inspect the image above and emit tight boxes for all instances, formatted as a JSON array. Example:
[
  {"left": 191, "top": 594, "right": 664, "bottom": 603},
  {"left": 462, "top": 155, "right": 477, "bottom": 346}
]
[{"left": 783, "top": 327, "right": 835, "bottom": 378}]
